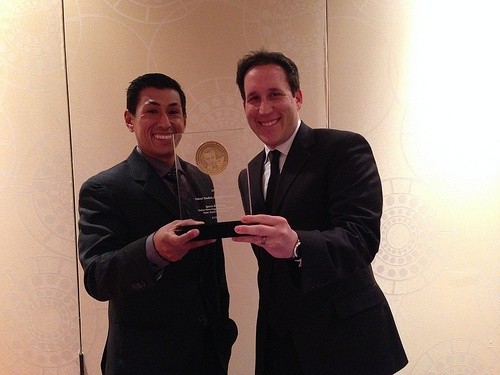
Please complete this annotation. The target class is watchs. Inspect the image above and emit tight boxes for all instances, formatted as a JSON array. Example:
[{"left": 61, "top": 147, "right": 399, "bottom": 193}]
[{"left": 291, "top": 235, "right": 303, "bottom": 260}]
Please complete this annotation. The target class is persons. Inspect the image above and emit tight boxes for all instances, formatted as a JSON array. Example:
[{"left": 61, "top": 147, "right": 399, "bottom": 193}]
[
  {"left": 80, "top": 73, "right": 239, "bottom": 375},
  {"left": 232, "top": 46, "right": 408, "bottom": 375}
]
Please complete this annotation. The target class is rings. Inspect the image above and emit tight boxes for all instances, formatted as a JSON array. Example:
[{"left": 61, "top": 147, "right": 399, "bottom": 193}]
[{"left": 260, "top": 236, "right": 266, "bottom": 244}]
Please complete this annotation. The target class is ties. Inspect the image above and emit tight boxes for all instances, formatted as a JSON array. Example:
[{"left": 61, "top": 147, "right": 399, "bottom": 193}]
[
  {"left": 264, "top": 149, "right": 283, "bottom": 215},
  {"left": 165, "top": 172, "right": 200, "bottom": 222}
]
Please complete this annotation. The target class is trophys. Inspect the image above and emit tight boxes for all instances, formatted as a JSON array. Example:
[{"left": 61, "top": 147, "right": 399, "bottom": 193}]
[{"left": 172, "top": 127, "right": 252, "bottom": 241}]
